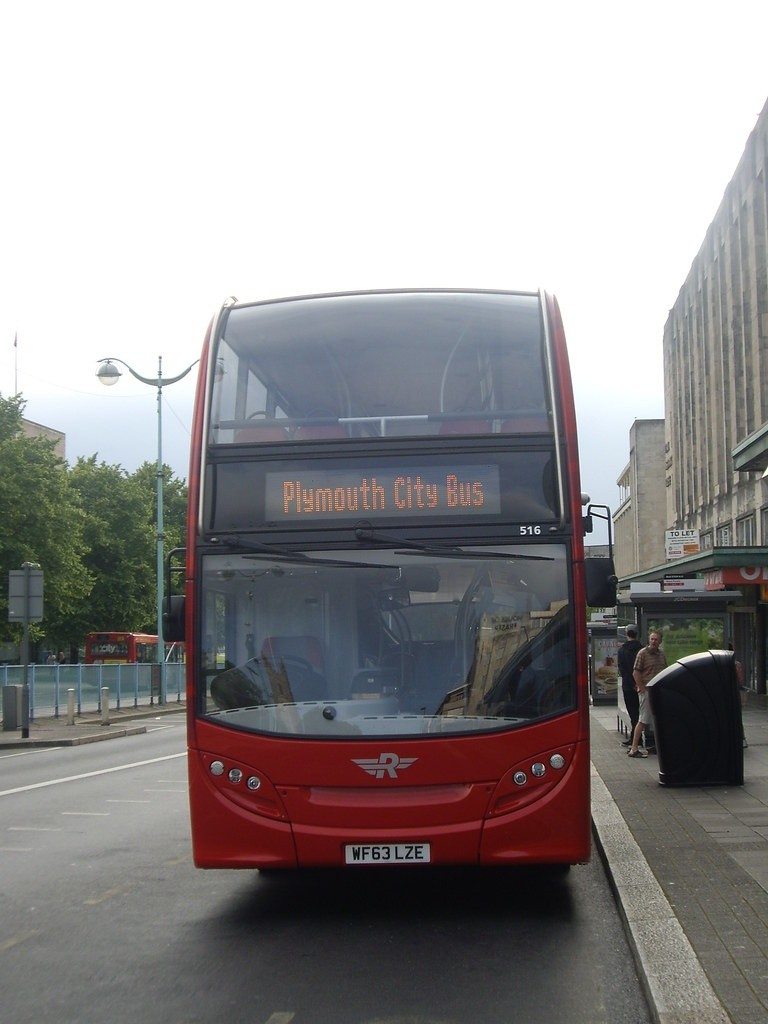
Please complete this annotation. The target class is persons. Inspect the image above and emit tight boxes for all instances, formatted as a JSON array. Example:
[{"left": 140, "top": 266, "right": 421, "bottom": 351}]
[
  {"left": 70, "top": 645, "right": 80, "bottom": 664},
  {"left": 46, "top": 650, "right": 56, "bottom": 666},
  {"left": 57, "top": 650, "right": 66, "bottom": 664},
  {"left": 626, "top": 631, "right": 669, "bottom": 758},
  {"left": 616, "top": 623, "right": 643, "bottom": 748},
  {"left": 727, "top": 643, "right": 749, "bottom": 748}
]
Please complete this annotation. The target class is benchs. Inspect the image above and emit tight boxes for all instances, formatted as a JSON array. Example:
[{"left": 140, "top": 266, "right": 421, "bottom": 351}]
[{"left": 616, "top": 710, "right": 656, "bottom": 751}]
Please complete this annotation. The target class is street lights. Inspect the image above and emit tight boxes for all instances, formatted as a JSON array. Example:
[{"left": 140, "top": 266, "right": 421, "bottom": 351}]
[{"left": 95, "top": 355, "right": 200, "bottom": 705}]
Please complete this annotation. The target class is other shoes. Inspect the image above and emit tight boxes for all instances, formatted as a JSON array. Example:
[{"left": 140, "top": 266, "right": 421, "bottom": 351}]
[
  {"left": 622, "top": 740, "right": 633, "bottom": 746},
  {"left": 627, "top": 748, "right": 648, "bottom": 758}
]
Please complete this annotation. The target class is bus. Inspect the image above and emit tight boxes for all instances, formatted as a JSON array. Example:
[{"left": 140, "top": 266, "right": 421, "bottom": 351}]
[
  {"left": 85, "top": 631, "right": 185, "bottom": 693},
  {"left": 162, "top": 288, "right": 618, "bottom": 887}
]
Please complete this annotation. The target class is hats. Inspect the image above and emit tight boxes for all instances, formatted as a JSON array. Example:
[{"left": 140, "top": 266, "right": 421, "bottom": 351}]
[{"left": 625, "top": 624, "right": 638, "bottom": 635}]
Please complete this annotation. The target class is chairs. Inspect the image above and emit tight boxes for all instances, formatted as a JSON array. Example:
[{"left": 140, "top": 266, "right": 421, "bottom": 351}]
[
  {"left": 264, "top": 637, "right": 325, "bottom": 676},
  {"left": 437, "top": 420, "right": 550, "bottom": 434},
  {"left": 236, "top": 409, "right": 343, "bottom": 442}
]
